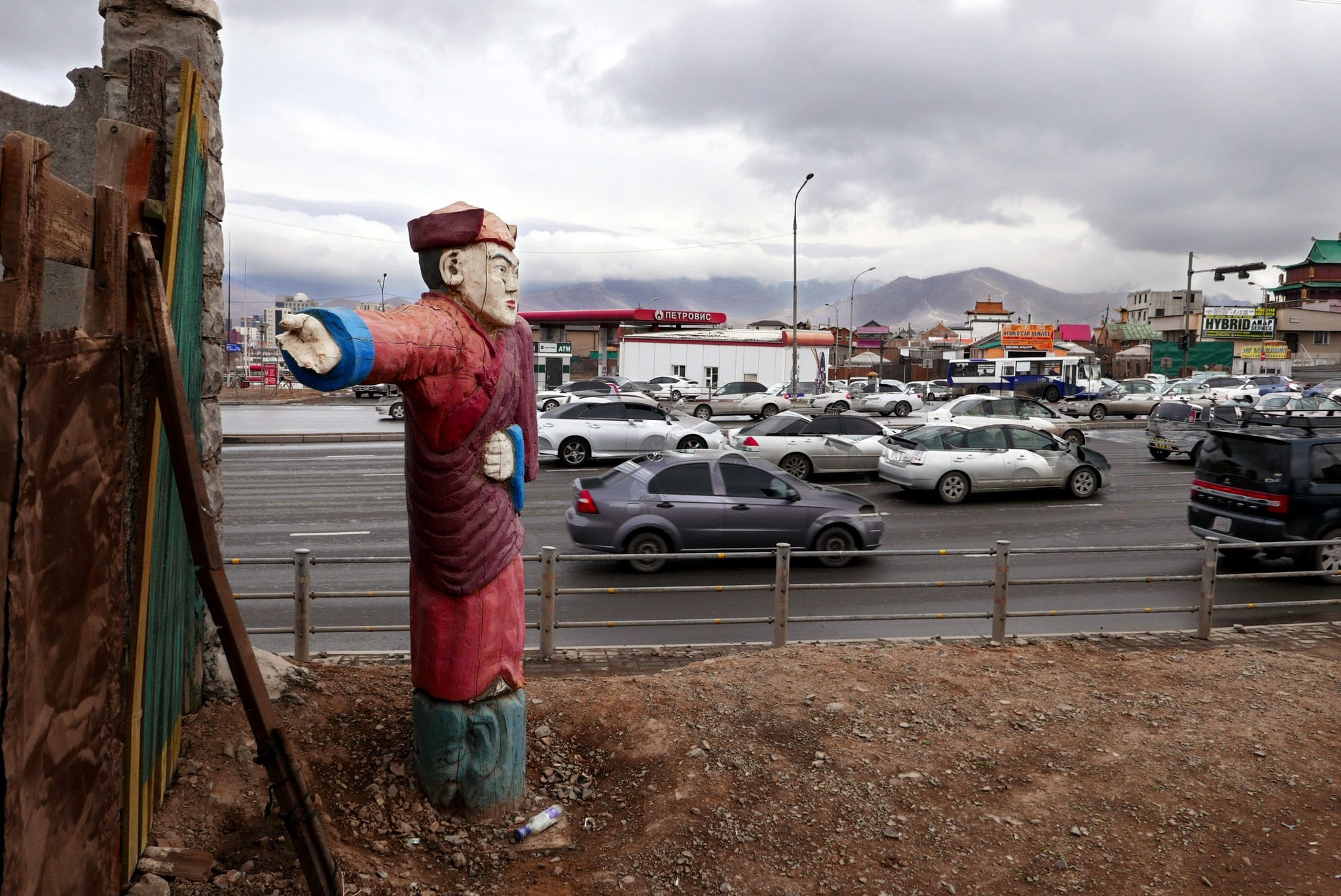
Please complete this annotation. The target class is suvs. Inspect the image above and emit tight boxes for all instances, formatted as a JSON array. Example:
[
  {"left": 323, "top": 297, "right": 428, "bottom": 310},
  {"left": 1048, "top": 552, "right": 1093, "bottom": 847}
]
[{"left": 1187, "top": 408, "right": 1341, "bottom": 586}]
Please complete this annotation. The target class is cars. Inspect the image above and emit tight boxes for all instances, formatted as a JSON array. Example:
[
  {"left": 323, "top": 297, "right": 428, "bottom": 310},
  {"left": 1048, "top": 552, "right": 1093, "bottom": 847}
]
[{"left": 357, "top": 370, "right": 1341, "bottom": 573}]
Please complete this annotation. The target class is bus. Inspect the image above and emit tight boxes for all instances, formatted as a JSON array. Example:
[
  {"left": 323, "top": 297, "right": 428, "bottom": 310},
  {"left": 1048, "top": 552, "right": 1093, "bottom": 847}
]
[{"left": 946, "top": 356, "right": 1101, "bottom": 402}]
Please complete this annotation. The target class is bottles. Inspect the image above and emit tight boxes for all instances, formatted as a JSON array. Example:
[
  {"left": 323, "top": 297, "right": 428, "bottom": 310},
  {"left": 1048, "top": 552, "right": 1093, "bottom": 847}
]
[{"left": 513, "top": 803, "right": 565, "bottom": 841}]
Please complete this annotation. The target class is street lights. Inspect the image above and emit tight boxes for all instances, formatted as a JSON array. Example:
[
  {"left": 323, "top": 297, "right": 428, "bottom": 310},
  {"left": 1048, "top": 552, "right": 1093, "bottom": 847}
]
[
  {"left": 791, "top": 173, "right": 876, "bottom": 399},
  {"left": 1247, "top": 281, "right": 1269, "bottom": 374},
  {"left": 378, "top": 273, "right": 387, "bottom": 312}
]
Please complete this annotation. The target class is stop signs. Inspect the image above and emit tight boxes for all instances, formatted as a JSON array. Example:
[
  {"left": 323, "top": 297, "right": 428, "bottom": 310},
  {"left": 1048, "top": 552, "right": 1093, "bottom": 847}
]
[{"left": 1261, "top": 352, "right": 1266, "bottom": 361}]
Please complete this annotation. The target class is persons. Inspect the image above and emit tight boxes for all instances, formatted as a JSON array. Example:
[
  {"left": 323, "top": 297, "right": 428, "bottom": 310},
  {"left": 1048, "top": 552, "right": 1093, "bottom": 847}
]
[
  {"left": 277, "top": 199, "right": 542, "bottom": 822},
  {"left": 957, "top": 363, "right": 1113, "bottom": 380}
]
